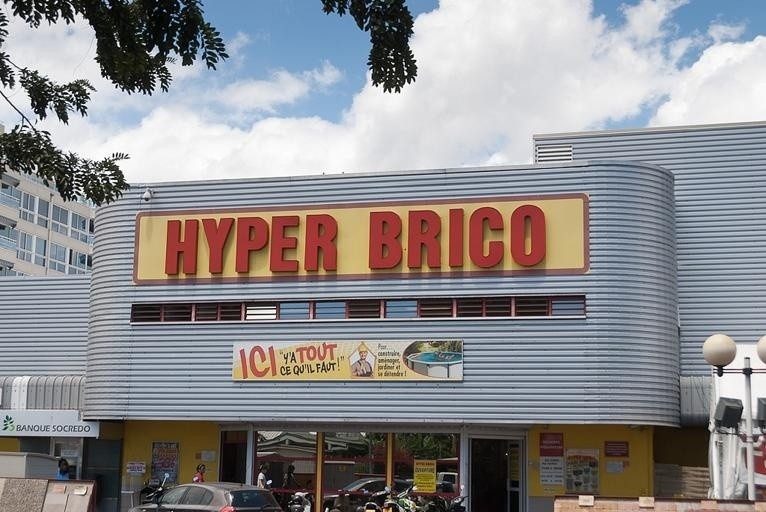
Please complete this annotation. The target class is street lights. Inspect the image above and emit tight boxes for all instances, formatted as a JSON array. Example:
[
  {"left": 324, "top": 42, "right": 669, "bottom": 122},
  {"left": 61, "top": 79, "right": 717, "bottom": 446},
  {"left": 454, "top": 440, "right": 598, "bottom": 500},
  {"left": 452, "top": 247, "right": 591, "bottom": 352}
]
[{"left": 702, "top": 332, "right": 766, "bottom": 500}]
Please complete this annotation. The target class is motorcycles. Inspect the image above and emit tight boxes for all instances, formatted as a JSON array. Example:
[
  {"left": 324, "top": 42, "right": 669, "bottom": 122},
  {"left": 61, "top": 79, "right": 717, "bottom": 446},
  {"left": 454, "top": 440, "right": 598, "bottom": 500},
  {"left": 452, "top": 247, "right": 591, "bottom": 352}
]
[
  {"left": 287, "top": 486, "right": 469, "bottom": 512},
  {"left": 143, "top": 471, "right": 170, "bottom": 503}
]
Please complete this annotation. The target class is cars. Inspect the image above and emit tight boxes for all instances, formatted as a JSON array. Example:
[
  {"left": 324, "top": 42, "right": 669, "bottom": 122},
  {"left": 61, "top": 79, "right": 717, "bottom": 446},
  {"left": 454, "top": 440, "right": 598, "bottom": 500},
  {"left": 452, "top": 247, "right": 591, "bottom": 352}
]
[
  {"left": 342, "top": 472, "right": 458, "bottom": 494},
  {"left": 126, "top": 479, "right": 284, "bottom": 511}
]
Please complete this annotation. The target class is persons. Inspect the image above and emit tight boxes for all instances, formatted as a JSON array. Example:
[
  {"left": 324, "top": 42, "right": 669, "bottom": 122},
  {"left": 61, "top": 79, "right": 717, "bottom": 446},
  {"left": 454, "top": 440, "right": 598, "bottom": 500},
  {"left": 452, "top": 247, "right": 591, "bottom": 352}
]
[
  {"left": 351, "top": 346, "right": 373, "bottom": 376},
  {"left": 55, "top": 454, "right": 69, "bottom": 480},
  {"left": 256, "top": 463, "right": 270, "bottom": 495},
  {"left": 191, "top": 463, "right": 207, "bottom": 482},
  {"left": 282, "top": 464, "right": 302, "bottom": 490}
]
